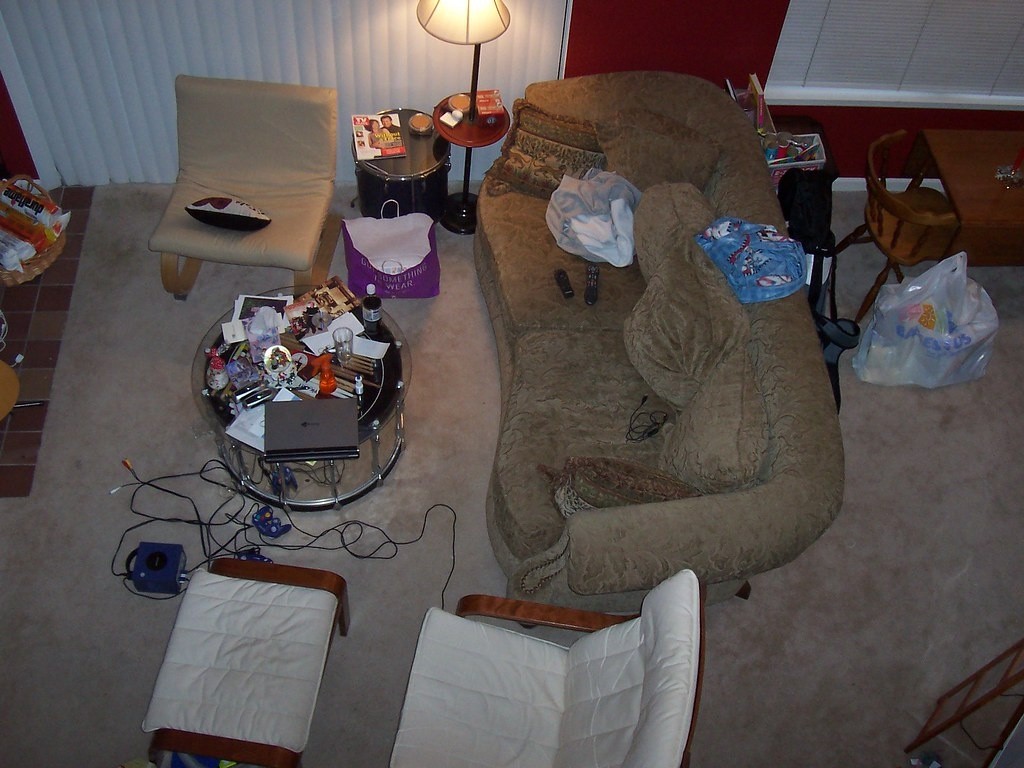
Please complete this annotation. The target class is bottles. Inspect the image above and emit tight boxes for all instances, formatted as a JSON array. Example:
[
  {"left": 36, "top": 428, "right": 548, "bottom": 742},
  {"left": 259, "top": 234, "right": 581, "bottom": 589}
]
[{"left": 362, "top": 284, "right": 383, "bottom": 334}]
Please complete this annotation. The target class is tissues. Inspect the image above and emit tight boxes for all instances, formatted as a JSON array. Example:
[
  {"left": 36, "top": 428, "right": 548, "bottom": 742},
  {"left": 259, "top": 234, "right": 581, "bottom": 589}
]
[{"left": 246, "top": 305, "right": 282, "bottom": 364}]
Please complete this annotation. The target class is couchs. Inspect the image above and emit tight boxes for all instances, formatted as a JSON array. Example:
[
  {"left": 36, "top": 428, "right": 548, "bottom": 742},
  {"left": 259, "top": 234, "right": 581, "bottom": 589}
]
[{"left": 469, "top": 67, "right": 847, "bottom": 624}]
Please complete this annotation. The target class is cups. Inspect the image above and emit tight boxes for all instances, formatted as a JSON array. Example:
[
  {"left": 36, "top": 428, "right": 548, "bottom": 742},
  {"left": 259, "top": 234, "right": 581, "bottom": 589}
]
[{"left": 331, "top": 327, "right": 355, "bottom": 363}]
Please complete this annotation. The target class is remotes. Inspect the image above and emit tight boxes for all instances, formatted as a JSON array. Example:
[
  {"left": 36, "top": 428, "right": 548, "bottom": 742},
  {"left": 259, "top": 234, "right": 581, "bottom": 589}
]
[{"left": 584, "top": 264, "right": 599, "bottom": 306}]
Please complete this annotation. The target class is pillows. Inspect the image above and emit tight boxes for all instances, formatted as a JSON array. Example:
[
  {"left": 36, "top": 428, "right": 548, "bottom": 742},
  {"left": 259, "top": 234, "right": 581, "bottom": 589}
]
[
  {"left": 618, "top": 234, "right": 754, "bottom": 413},
  {"left": 660, "top": 342, "right": 757, "bottom": 500},
  {"left": 491, "top": 94, "right": 608, "bottom": 198},
  {"left": 549, "top": 454, "right": 706, "bottom": 515},
  {"left": 596, "top": 108, "right": 721, "bottom": 209},
  {"left": 630, "top": 182, "right": 718, "bottom": 284}
]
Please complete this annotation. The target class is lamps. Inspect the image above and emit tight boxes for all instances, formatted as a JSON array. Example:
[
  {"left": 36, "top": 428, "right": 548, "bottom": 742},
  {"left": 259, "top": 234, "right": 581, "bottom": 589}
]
[{"left": 412, "top": 0, "right": 516, "bottom": 238}]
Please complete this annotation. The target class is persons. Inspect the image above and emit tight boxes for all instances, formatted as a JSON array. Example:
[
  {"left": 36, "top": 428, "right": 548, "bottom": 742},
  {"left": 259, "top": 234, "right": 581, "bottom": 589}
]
[
  {"left": 306, "top": 300, "right": 324, "bottom": 333},
  {"left": 250, "top": 305, "right": 262, "bottom": 313}
]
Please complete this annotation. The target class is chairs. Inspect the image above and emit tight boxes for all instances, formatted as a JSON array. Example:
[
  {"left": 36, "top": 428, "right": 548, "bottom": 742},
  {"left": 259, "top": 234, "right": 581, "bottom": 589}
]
[
  {"left": 387, "top": 565, "right": 709, "bottom": 768},
  {"left": 833, "top": 130, "right": 960, "bottom": 325},
  {"left": 145, "top": 71, "right": 348, "bottom": 298}
]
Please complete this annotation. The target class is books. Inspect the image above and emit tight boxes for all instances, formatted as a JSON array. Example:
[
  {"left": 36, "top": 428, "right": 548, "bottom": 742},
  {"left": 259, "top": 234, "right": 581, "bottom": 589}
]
[
  {"left": 351, "top": 114, "right": 408, "bottom": 161},
  {"left": 750, "top": 70, "right": 766, "bottom": 131},
  {"left": 284, "top": 298, "right": 329, "bottom": 341},
  {"left": 725, "top": 77, "right": 738, "bottom": 103}
]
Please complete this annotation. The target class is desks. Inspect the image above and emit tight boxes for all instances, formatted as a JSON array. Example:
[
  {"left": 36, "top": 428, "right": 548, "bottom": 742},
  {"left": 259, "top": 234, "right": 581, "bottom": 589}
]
[
  {"left": 352, "top": 90, "right": 453, "bottom": 237},
  {"left": 190, "top": 277, "right": 413, "bottom": 514},
  {"left": 903, "top": 122, "right": 1024, "bottom": 269}
]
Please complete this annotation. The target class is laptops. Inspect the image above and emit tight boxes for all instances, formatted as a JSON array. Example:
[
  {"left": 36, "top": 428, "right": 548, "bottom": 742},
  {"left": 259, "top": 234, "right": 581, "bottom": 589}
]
[{"left": 264, "top": 397, "right": 359, "bottom": 462}]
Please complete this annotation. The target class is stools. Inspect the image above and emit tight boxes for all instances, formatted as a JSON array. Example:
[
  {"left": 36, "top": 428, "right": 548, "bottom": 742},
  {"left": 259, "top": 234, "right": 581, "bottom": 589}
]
[{"left": 142, "top": 558, "right": 353, "bottom": 767}]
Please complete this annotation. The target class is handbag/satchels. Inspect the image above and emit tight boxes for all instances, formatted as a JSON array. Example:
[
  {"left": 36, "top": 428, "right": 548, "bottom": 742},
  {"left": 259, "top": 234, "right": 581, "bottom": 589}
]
[
  {"left": 340, "top": 198, "right": 440, "bottom": 299},
  {"left": 856, "top": 250, "right": 997, "bottom": 392}
]
